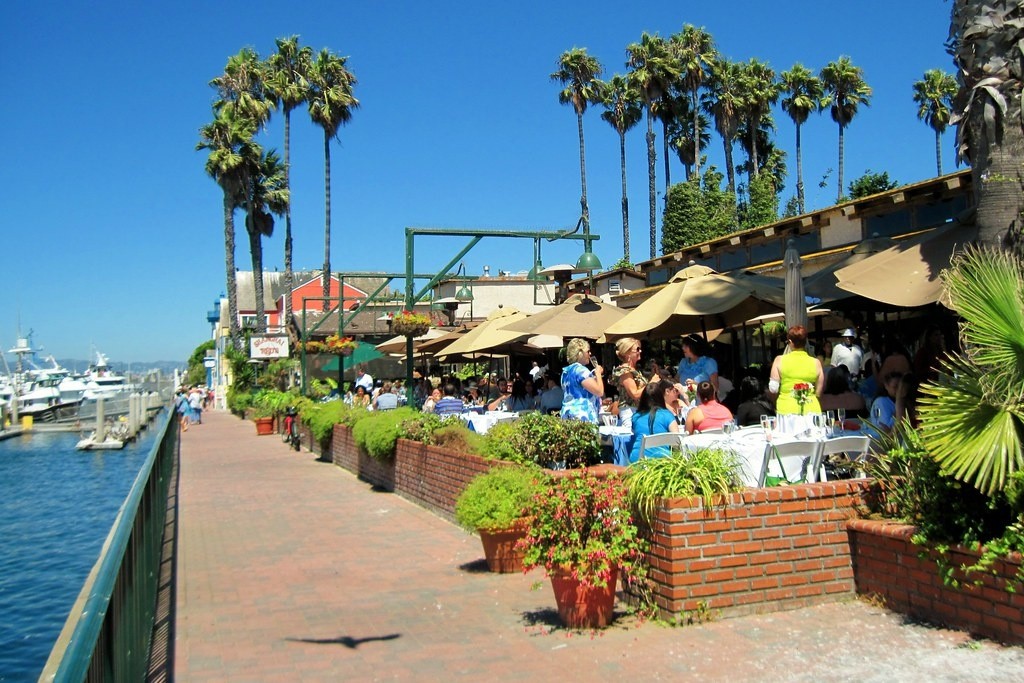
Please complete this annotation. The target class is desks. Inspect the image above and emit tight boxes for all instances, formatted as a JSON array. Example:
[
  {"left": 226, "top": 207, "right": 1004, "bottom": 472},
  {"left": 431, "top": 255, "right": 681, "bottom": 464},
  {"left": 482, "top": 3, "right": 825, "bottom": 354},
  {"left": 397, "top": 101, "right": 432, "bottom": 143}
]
[
  {"left": 443, "top": 411, "right": 517, "bottom": 434},
  {"left": 683, "top": 419, "right": 873, "bottom": 488},
  {"left": 598, "top": 425, "right": 633, "bottom": 445}
]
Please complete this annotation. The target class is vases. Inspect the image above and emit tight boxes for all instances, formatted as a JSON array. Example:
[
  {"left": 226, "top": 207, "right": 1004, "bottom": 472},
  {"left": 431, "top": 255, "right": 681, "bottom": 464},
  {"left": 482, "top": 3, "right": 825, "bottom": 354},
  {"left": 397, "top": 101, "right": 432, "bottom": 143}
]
[
  {"left": 395, "top": 324, "right": 428, "bottom": 336},
  {"left": 544, "top": 560, "right": 619, "bottom": 627},
  {"left": 336, "top": 347, "right": 355, "bottom": 356},
  {"left": 305, "top": 346, "right": 318, "bottom": 354}
]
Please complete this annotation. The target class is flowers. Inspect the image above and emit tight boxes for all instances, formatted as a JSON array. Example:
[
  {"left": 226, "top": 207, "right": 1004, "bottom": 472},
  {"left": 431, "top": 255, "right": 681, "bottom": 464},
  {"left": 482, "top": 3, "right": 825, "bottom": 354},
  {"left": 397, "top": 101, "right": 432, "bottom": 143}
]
[
  {"left": 296, "top": 333, "right": 360, "bottom": 351},
  {"left": 791, "top": 383, "right": 816, "bottom": 414},
  {"left": 513, "top": 462, "right": 650, "bottom": 590},
  {"left": 685, "top": 385, "right": 696, "bottom": 404},
  {"left": 389, "top": 308, "right": 432, "bottom": 327}
]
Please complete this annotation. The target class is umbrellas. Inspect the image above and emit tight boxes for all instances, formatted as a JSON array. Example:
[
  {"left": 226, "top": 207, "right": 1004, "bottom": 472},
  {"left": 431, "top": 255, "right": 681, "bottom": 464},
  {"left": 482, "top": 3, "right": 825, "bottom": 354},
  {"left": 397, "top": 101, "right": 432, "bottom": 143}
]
[
  {"left": 321, "top": 340, "right": 385, "bottom": 372},
  {"left": 376, "top": 203, "right": 981, "bottom": 404}
]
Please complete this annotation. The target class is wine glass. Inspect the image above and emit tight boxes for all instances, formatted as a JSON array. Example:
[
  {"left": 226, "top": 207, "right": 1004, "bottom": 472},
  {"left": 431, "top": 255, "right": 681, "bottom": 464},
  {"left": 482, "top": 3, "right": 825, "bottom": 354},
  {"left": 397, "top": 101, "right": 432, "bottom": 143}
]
[
  {"left": 677, "top": 408, "right": 684, "bottom": 429},
  {"left": 874, "top": 404, "right": 881, "bottom": 426},
  {"left": 611, "top": 415, "right": 618, "bottom": 432},
  {"left": 760, "top": 410, "right": 836, "bottom": 440},
  {"left": 722, "top": 419, "right": 735, "bottom": 443},
  {"left": 590, "top": 356, "right": 604, "bottom": 377},
  {"left": 838, "top": 408, "right": 845, "bottom": 434}
]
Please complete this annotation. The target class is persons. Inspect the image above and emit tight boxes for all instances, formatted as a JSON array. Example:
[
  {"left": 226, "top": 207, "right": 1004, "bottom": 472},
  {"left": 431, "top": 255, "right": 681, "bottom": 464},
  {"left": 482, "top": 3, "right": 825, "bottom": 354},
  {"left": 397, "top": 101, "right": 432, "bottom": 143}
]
[
  {"left": 329, "top": 323, "right": 948, "bottom": 471},
  {"left": 174, "top": 385, "right": 212, "bottom": 432}
]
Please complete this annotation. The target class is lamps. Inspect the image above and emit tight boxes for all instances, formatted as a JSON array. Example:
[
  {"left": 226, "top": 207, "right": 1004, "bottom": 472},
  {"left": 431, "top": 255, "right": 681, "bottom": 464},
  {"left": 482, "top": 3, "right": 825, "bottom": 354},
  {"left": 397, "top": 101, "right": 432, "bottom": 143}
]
[
  {"left": 546, "top": 216, "right": 603, "bottom": 269},
  {"left": 527, "top": 237, "right": 550, "bottom": 282},
  {"left": 450, "top": 263, "right": 475, "bottom": 301}
]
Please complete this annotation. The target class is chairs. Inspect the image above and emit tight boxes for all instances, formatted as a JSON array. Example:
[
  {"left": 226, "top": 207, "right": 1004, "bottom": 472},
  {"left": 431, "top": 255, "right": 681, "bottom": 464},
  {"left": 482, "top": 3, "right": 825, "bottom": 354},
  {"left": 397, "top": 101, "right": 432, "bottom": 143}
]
[
  {"left": 641, "top": 424, "right": 728, "bottom": 462},
  {"left": 758, "top": 434, "right": 872, "bottom": 487}
]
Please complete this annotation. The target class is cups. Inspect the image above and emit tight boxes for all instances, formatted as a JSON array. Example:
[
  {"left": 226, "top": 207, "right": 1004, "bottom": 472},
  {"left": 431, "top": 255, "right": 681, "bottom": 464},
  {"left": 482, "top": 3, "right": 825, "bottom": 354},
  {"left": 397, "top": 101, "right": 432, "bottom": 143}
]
[
  {"left": 478, "top": 397, "right": 483, "bottom": 406},
  {"left": 472, "top": 399, "right": 476, "bottom": 408}
]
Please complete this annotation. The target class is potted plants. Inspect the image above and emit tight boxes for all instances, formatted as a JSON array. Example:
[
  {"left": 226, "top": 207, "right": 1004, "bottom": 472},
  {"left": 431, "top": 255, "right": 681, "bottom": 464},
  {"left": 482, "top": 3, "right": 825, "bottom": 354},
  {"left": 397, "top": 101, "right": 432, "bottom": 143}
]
[
  {"left": 454, "top": 463, "right": 545, "bottom": 574},
  {"left": 251, "top": 408, "right": 276, "bottom": 436}
]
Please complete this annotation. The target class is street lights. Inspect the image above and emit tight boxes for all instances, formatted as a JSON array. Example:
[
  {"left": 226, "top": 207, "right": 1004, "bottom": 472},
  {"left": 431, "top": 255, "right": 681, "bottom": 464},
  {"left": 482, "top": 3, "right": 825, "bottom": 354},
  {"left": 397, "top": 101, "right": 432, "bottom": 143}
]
[
  {"left": 298, "top": 295, "right": 407, "bottom": 401},
  {"left": 403, "top": 215, "right": 603, "bottom": 414},
  {"left": 337, "top": 262, "right": 481, "bottom": 400}
]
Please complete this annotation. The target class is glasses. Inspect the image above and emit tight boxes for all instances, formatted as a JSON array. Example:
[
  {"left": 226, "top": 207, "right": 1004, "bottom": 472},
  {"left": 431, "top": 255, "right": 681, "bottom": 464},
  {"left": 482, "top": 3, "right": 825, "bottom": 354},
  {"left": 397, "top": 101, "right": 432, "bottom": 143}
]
[
  {"left": 586, "top": 350, "right": 590, "bottom": 353},
  {"left": 630, "top": 347, "right": 642, "bottom": 352},
  {"left": 785, "top": 340, "right": 789, "bottom": 344}
]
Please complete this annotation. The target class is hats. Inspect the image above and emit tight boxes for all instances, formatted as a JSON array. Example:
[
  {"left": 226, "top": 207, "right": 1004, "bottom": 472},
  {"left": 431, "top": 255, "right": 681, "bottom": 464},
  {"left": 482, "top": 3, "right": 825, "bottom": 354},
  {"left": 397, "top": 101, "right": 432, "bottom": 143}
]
[
  {"left": 464, "top": 381, "right": 478, "bottom": 391},
  {"left": 841, "top": 329, "right": 855, "bottom": 337}
]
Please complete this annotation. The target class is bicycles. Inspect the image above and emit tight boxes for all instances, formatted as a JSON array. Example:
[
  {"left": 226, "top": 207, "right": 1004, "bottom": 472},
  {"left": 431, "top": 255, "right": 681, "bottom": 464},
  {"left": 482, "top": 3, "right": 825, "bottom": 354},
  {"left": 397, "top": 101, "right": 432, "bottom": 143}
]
[{"left": 281, "top": 400, "right": 304, "bottom": 452}]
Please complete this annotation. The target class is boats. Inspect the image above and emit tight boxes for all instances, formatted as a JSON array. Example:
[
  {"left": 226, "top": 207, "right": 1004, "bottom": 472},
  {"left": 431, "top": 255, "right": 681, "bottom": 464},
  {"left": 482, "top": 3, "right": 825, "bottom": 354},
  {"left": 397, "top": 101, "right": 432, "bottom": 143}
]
[{"left": 0, "top": 314, "right": 146, "bottom": 441}]
